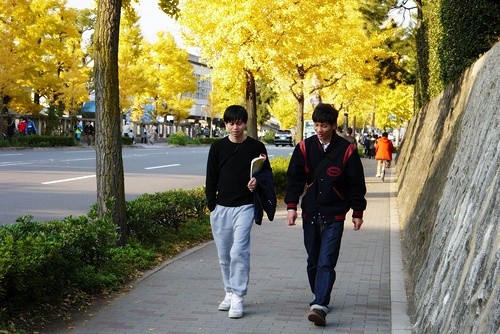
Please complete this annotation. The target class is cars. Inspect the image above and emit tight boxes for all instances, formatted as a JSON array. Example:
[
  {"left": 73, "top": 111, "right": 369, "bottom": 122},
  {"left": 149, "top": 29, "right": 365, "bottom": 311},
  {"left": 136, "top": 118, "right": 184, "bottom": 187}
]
[{"left": 273, "top": 129, "right": 294, "bottom": 147}]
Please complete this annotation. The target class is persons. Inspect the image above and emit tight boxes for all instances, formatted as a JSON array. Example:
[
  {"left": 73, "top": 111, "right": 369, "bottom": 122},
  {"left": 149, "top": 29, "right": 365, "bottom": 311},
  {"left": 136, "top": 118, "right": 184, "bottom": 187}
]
[
  {"left": 8, "top": 113, "right": 161, "bottom": 148},
  {"left": 284, "top": 103, "right": 367, "bottom": 327},
  {"left": 194, "top": 122, "right": 267, "bottom": 139},
  {"left": 338, "top": 122, "right": 397, "bottom": 183},
  {"left": 205, "top": 105, "right": 276, "bottom": 318}
]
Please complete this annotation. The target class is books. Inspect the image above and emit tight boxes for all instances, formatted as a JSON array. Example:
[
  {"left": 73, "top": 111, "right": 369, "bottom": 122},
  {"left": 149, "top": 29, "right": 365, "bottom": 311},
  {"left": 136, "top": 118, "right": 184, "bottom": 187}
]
[{"left": 250, "top": 154, "right": 266, "bottom": 178}]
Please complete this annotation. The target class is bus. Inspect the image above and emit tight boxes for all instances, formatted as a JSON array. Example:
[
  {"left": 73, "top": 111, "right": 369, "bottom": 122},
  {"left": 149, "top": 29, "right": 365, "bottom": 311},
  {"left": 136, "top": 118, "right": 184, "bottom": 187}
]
[{"left": 303, "top": 120, "right": 317, "bottom": 140}]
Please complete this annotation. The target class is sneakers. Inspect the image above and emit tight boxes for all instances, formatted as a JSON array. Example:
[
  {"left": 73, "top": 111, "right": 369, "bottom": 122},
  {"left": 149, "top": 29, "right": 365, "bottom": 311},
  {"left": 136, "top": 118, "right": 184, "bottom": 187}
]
[{"left": 218, "top": 292, "right": 244, "bottom": 317}]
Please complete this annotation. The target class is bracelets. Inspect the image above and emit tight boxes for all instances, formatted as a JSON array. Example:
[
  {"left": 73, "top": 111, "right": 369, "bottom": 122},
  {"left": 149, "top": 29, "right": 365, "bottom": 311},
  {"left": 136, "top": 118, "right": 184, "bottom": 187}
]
[{"left": 287, "top": 208, "right": 295, "bottom": 212}]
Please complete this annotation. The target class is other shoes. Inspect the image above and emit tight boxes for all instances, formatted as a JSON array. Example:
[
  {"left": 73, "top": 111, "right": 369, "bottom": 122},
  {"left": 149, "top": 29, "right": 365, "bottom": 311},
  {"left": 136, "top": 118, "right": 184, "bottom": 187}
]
[
  {"left": 376, "top": 174, "right": 380, "bottom": 178},
  {"left": 307, "top": 308, "right": 327, "bottom": 327}
]
